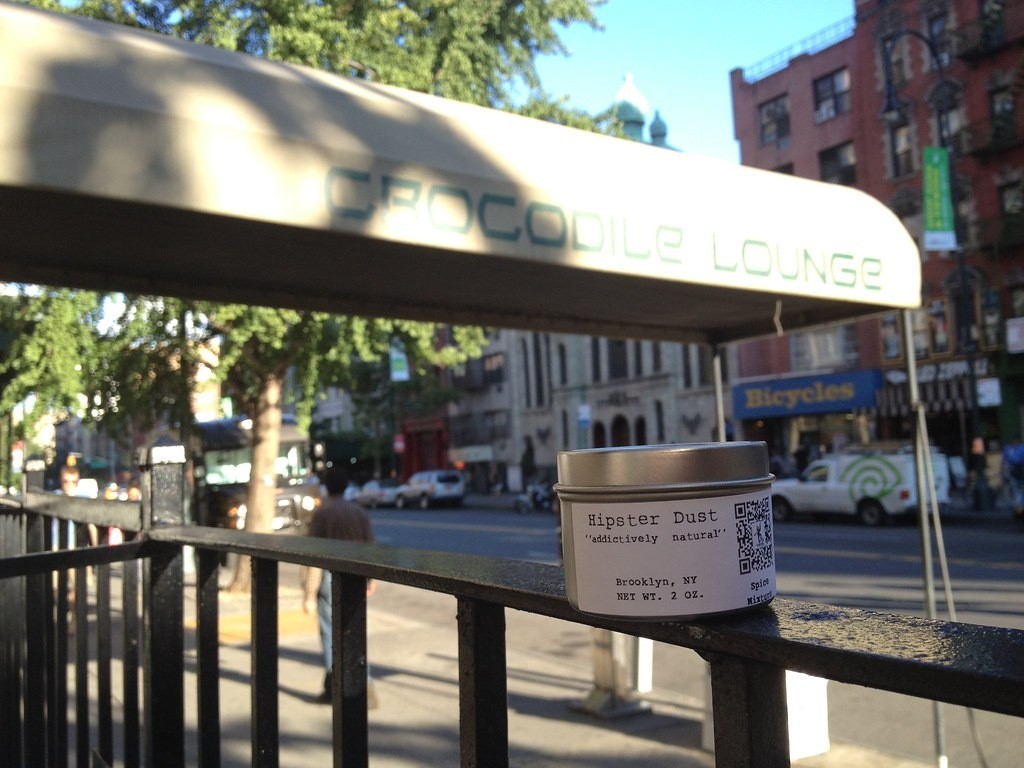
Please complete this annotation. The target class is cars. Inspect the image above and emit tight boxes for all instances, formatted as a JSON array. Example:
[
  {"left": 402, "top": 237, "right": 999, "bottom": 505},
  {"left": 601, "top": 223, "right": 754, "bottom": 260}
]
[{"left": 353, "top": 478, "right": 400, "bottom": 511}]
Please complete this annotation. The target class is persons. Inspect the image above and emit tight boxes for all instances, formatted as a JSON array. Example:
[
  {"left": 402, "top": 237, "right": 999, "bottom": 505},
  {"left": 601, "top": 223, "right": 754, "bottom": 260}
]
[
  {"left": 105, "top": 486, "right": 122, "bottom": 545},
  {"left": 769, "top": 442, "right": 809, "bottom": 477},
  {"left": 968, "top": 437, "right": 996, "bottom": 512},
  {"left": 126, "top": 486, "right": 140, "bottom": 501},
  {"left": 301, "top": 488, "right": 379, "bottom": 709},
  {"left": 50, "top": 466, "right": 98, "bottom": 606},
  {"left": 1002, "top": 433, "right": 1024, "bottom": 487}
]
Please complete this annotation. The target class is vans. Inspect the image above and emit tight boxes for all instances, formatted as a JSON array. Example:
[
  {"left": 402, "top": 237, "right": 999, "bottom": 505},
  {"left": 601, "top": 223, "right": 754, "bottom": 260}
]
[{"left": 192, "top": 419, "right": 329, "bottom": 540}]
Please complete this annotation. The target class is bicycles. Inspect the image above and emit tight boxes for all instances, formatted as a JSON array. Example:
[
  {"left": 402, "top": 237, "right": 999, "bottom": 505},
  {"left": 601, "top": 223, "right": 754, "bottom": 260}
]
[{"left": 961, "top": 462, "right": 1022, "bottom": 512}]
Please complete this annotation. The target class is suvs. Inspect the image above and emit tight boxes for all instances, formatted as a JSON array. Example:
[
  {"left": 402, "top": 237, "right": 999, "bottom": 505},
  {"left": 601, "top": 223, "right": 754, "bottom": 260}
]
[
  {"left": 394, "top": 469, "right": 467, "bottom": 510},
  {"left": 769, "top": 448, "right": 954, "bottom": 528}
]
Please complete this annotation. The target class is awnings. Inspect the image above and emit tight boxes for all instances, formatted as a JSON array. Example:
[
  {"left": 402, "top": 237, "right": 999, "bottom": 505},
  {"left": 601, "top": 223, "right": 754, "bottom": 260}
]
[{"left": 0, "top": 0, "right": 988, "bottom": 768}]
